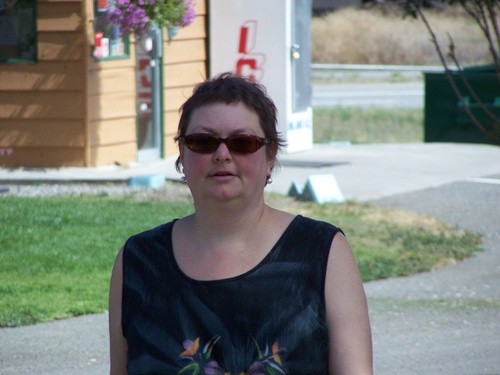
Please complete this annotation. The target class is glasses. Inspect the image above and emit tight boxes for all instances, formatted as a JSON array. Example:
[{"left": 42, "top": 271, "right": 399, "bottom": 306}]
[{"left": 181, "top": 132, "right": 271, "bottom": 155}]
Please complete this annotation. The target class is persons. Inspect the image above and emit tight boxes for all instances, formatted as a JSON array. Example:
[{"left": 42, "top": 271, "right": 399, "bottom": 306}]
[{"left": 109, "top": 73, "right": 373, "bottom": 375}]
